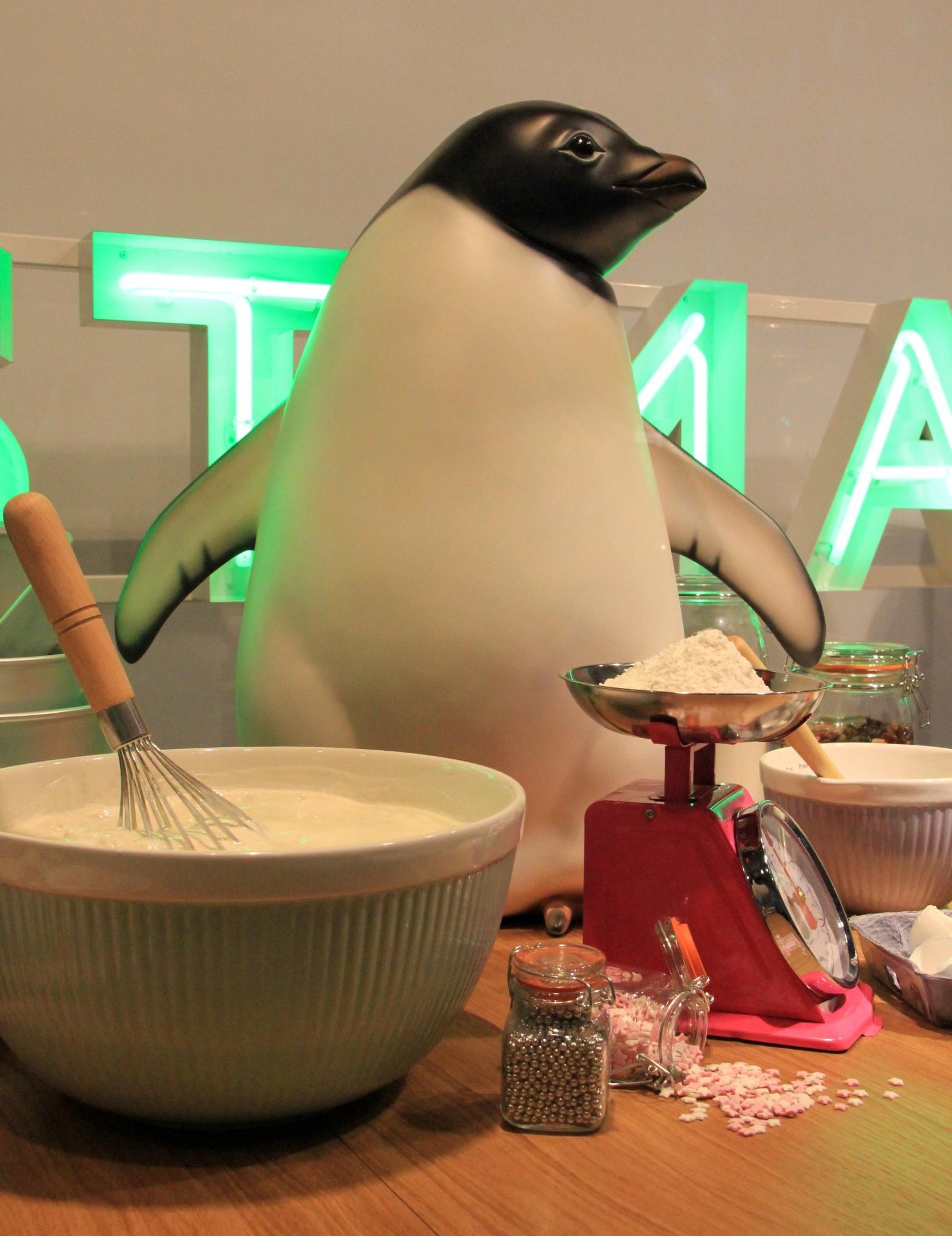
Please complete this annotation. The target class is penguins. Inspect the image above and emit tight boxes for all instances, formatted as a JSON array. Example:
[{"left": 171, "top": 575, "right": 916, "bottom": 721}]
[{"left": 111, "top": 100, "right": 828, "bottom": 937}]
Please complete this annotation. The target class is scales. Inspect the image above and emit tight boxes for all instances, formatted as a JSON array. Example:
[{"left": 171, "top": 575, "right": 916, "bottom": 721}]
[{"left": 558, "top": 662, "right": 882, "bottom": 1051}]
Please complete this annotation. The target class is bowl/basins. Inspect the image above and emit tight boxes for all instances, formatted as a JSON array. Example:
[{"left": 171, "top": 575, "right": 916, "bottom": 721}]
[
  {"left": 0, "top": 743, "right": 525, "bottom": 1123},
  {"left": 761, "top": 741, "right": 951, "bottom": 913},
  {"left": 848, "top": 911, "right": 952, "bottom": 1030}
]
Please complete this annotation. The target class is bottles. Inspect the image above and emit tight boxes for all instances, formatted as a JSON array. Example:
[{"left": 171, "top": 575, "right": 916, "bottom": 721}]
[
  {"left": 782, "top": 640, "right": 931, "bottom": 744},
  {"left": 500, "top": 941, "right": 616, "bottom": 1135},
  {"left": 602, "top": 917, "right": 714, "bottom": 1095},
  {"left": 675, "top": 573, "right": 768, "bottom": 672}
]
